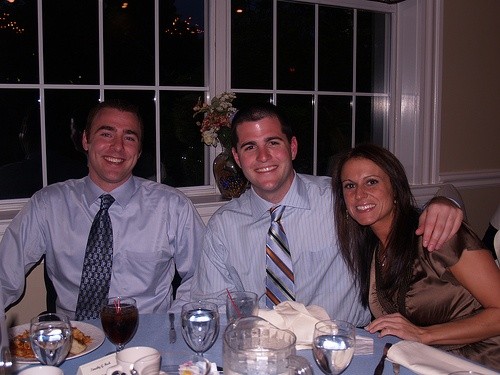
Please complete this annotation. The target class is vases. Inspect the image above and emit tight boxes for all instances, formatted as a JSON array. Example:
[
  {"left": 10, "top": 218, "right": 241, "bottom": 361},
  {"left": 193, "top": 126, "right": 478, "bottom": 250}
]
[{"left": 213, "top": 128, "right": 249, "bottom": 201}]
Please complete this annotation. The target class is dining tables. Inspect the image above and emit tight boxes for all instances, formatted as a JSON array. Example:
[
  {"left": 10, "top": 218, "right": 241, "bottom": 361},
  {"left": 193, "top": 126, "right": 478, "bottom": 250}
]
[{"left": 0, "top": 286, "right": 500, "bottom": 375}]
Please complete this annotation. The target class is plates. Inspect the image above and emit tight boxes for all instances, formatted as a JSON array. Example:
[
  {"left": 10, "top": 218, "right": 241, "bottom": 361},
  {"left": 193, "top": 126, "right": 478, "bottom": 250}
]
[{"left": 6, "top": 320, "right": 106, "bottom": 364}]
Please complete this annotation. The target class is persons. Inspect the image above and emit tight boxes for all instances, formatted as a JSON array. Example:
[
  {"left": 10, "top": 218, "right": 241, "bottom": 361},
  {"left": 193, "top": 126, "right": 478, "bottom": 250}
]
[
  {"left": 190, "top": 102, "right": 464, "bottom": 327},
  {"left": 332, "top": 145, "right": 500, "bottom": 370},
  {"left": 0, "top": 101, "right": 206, "bottom": 321}
]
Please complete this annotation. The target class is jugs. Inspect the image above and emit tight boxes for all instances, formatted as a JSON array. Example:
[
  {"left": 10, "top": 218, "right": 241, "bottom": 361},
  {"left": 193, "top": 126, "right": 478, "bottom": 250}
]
[{"left": 223, "top": 315, "right": 314, "bottom": 374}]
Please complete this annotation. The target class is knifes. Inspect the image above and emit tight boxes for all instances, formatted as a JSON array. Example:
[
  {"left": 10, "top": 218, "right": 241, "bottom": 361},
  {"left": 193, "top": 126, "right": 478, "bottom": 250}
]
[
  {"left": 392, "top": 363, "right": 400, "bottom": 375},
  {"left": 373, "top": 342, "right": 392, "bottom": 375},
  {"left": 168, "top": 312, "right": 177, "bottom": 344}
]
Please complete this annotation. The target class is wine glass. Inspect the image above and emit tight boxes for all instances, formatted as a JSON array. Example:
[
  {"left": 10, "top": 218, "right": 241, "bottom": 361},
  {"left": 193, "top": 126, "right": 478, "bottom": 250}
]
[
  {"left": 179, "top": 301, "right": 221, "bottom": 363},
  {"left": 100, "top": 296, "right": 140, "bottom": 356}
]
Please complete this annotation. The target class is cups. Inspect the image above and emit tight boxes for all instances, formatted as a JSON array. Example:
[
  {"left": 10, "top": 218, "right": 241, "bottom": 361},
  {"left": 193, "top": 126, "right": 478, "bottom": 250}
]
[
  {"left": 17, "top": 365, "right": 64, "bottom": 375},
  {"left": 311, "top": 320, "right": 356, "bottom": 375},
  {"left": 227, "top": 291, "right": 258, "bottom": 325},
  {"left": 30, "top": 313, "right": 73, "bottom": 366},
  {"left": 449, "top": 370, "right": 485, "bottom": 375},
  {"left": 107, "top": 346, "right": 161, "bottom": 375},
  {"left": 134, "top": 352, "right": 212, "bottom": 375}
]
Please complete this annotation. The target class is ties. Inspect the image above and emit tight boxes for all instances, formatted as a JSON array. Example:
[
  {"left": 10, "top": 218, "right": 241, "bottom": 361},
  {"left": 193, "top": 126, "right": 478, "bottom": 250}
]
[
  {"left": 265, "top": 204, "right": 295, "bottom": 310},
  {"left": 74, "top": 194, "right": 115, "bottom": 321}
]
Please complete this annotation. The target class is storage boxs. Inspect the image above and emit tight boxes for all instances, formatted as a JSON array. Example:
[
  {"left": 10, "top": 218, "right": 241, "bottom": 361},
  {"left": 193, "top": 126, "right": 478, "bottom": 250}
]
[{"left": 387, "top": 337, "right": 500, "bottom": 375}]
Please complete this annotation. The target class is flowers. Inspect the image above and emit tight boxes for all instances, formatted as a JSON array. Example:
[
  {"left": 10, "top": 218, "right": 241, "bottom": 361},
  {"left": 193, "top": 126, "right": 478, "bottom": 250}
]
[{"left": 192, "top": 92, "right": 238, "bottom": 148}]
